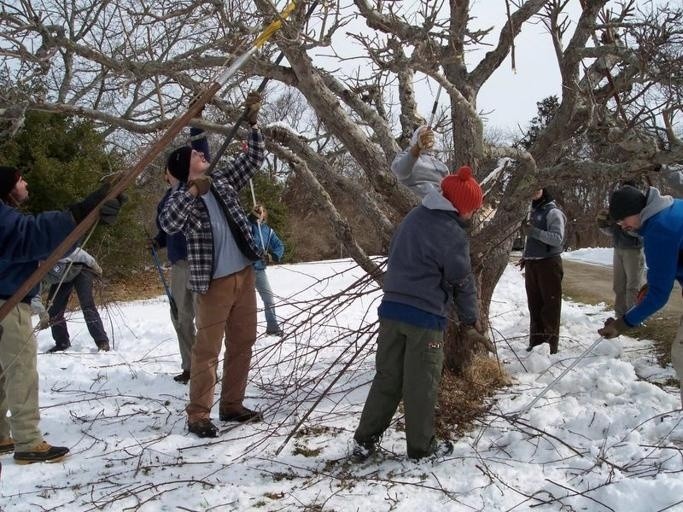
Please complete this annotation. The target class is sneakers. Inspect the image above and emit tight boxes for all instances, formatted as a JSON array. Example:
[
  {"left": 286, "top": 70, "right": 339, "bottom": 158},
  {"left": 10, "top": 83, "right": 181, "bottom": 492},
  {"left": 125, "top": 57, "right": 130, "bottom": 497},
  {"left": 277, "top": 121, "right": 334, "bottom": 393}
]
[
  {"left": 97, "top": 340, "right": 110, "bottom": 352},
  {"left": 218, "top": 406, "right": 264, "bottom": 423},
  {"left": 173, "top": 370, "right": 191, "bottom": 384},
  {"left": 407, "top": 441, "right": 454, "bottom": 465},
  {"left": 14, "top": 441, "right": 70, "bottom": 465},
  {"left": 349, "top": 444, "right": 373, "bottom": 463},
  {"left": 46, "top": 342, "right": 71, "bottom": 355},
  {"left": 187, "top": 419, "right": 220, "bottom": 438},
  {"left": 0, "top": 440, "right": 15, "bottom": 455}
]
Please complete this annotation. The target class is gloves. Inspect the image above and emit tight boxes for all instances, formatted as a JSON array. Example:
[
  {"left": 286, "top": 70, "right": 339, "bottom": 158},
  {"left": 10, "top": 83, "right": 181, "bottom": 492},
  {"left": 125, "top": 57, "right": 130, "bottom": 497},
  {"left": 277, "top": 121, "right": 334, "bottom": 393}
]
[
  {"left": 186, "top": 176, "right": 211, "bottom": 196},
  {"left": 244, "top": 89, "right": 263, "bottom": 126},
  {"left": 71, "top": 182, "right": 128, "bottom": 230}
]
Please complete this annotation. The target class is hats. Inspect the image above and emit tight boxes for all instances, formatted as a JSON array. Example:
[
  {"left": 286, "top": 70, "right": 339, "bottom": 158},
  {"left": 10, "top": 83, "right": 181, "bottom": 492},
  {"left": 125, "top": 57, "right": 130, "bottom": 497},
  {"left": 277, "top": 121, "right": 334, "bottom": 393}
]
[
  {"left": 608, "top": 185, "right": 649, "bottom": 225},
  {"left": 441, "top": 166, "right": 483, "bottom": 214},
  {"left": 166, "top": 146, "right": 192, "bottom": 182}
]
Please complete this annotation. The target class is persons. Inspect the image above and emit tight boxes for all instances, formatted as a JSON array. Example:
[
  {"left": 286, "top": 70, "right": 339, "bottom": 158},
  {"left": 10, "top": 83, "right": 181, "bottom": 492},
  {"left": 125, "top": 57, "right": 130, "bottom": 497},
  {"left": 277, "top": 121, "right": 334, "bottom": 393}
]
[
  {"left": 140, "top": 163, "right": 196, "bottom": 385},
  {"left": 387, "top": 122, "right": 451, "bottom": 202},
  {"left": 243, "top": 202, "right": 289, "bottom": 340},
  {"left": 155, "top": 89, "right": 266, "bottom": 439},
  {"left": 29, "top": 243, "right": 111, "bottom": 356},
  {"left": 597, "top": 182, "right": 683, "bottom": 343},
  {"left": 0, "top": 179, "right": 130, "bottom": 267},
  {"left": 0, "top": 162, "right": 70, "bottom": 466},
  {"left": 516, "top": 183, "right": 569, "bottom": 355},
  {"left": 605, "top": 178, "right": 644, "bottom": 322},
  {"left": 346, "top": 163, "right": 487, "bottom": 465}
]
